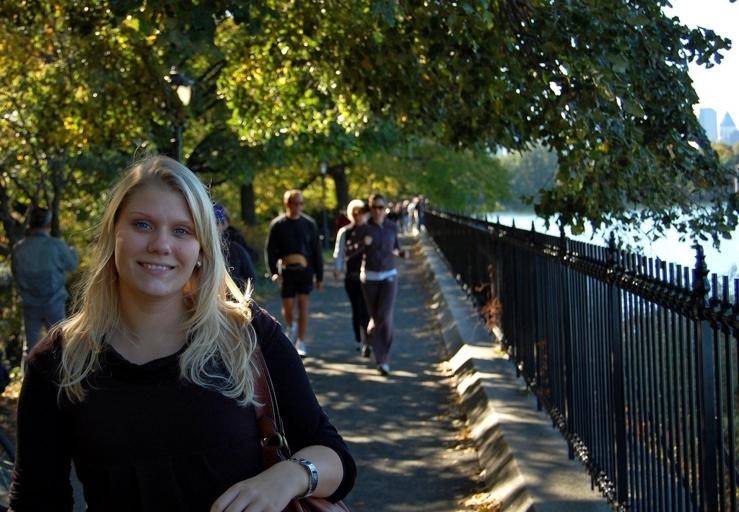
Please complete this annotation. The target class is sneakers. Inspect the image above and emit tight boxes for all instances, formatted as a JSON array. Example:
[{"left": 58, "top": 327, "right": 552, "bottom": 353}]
[
  {"left": 355, "top": 342, "right": 390, "bottom": 375},
  {"left": 286, "top": 322, "right": 307, "bottom": 358}
]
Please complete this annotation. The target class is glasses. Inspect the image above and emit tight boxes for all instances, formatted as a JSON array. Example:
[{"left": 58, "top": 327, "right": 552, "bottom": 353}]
[{"left": 372, "top": 205, "right": 383, "bottom": 209}]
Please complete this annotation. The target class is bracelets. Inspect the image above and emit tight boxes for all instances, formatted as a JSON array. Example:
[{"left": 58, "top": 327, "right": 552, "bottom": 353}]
[{"left": 288, "top": 459, "right": 318, "bottom": 501}]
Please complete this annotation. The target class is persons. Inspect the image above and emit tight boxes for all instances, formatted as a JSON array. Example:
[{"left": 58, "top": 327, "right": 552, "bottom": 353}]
[
  {"left": 330, "top": 189, "right": 431, "bottom": 375},
  {"left": 264, "top": 189, "right": 324, "bottom": 360},
  {"left": 220, "top": 206, "right": 259, "bottom": 263},
  {"left": 7, "top": 154, "right": 357, "bottom": 511},
  {"left": 213, "top": 205, "right": 257, "bottom": 302},
  {"left": 14, "top": 206, "right": 78, "bottom": 354}
]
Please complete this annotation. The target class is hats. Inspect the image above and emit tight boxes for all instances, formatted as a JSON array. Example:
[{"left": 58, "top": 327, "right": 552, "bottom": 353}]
[{"left": 213, "top": 205, "right": 225, "bottom": 222}]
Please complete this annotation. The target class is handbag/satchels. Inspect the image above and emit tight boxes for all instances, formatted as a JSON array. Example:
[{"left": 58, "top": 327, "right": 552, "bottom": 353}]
[
  {"left": 262, "top": 444, "right": 351, "bottom": 511},
  {"left": 281, "top": 252, "right": 312, "bottom": 272}
]
